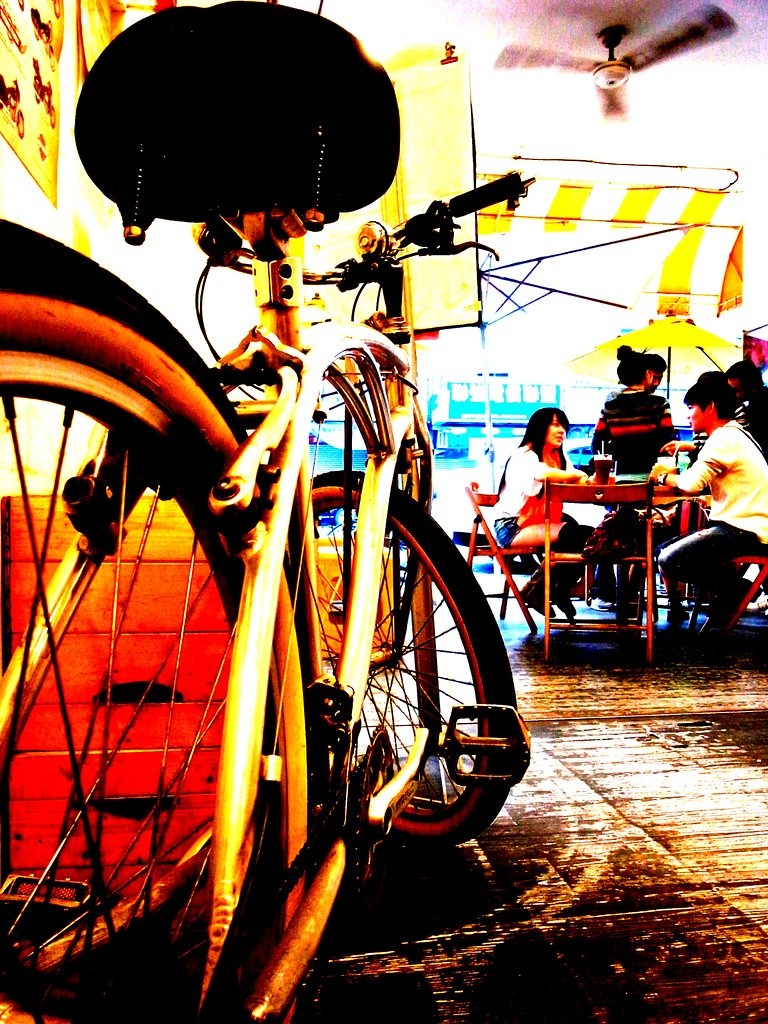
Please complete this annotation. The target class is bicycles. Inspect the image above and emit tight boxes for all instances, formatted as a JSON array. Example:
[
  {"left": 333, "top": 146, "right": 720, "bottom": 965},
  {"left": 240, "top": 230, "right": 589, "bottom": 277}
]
[{"left": 0, "top": 1, "right": 533, "bottom": 1024}]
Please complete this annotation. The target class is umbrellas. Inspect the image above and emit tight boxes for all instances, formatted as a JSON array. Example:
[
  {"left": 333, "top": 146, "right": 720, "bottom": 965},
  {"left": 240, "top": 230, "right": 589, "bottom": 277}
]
[{"left": 565, "top": 310, "right": 741, "bottom": 399}]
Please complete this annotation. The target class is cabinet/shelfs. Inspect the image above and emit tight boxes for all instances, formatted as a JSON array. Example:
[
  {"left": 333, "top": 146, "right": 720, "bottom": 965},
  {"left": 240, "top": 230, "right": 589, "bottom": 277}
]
[{"left": 0, "top": 495, "right": 234, "bottom": 895}]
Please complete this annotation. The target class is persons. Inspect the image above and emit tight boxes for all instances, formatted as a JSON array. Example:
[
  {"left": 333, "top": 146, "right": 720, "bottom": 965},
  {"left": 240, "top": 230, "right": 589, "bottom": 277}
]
[
  {"left": 494, "top": 408, "right": 596, "bottom": 617},
  {"left": 647, "top": 359, "right": 768, "bottom": 617},
  {"left": 589, "top": 346, "right": 690, "bottom": 623}
]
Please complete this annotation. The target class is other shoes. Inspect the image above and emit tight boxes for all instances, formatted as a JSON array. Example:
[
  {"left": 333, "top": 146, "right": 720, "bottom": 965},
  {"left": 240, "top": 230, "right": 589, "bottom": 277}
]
[
  {"left": 591, "top": 596, "right": 613, "bottom": 613},
  {"left": 717, "top": 562, "right": 737, "bottom": 608},
  {"left": 520, "top": 583, "right": 555, "bottom": 618},
  {"left": 550, "top": 588, "right": 576, "bottom": 616}
]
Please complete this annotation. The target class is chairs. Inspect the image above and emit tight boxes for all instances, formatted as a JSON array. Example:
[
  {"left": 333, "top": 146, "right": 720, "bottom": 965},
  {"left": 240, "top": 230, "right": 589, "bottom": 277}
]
[
  {"left": 542, "top": 482, "right": 768, "bottom": 663},
  {"left": 465, "top": 486, "right": 576, "bottom": 635}
]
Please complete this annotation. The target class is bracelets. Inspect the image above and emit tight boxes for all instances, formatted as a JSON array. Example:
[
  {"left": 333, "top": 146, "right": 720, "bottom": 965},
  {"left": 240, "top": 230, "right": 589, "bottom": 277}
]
[{"left": 657, "top": 473, "right": 668, "bottom": 484}]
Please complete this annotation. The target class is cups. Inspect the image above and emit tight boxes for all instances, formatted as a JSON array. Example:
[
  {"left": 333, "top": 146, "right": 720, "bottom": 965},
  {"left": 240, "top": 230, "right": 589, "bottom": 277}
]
[
  {"left": 608, "top": 461, "right": 617, "bottom": 484},
  {"left": 658, "top": 457, "right": 677, "bottom": 475},
  {"left": 594, "top": 454, "right": 612, "bottom": 484}
]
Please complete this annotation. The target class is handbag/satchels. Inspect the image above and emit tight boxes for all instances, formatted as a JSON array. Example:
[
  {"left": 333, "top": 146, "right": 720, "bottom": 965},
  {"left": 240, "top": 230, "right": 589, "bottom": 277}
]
[{"left": 582, "top": 505, "right": 638, "bottom": 563}]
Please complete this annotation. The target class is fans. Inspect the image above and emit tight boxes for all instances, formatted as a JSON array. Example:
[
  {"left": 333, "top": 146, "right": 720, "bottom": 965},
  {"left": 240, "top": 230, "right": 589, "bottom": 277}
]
[{"left": 494, "top": 3, "right": 739, "bottom": 122}]
